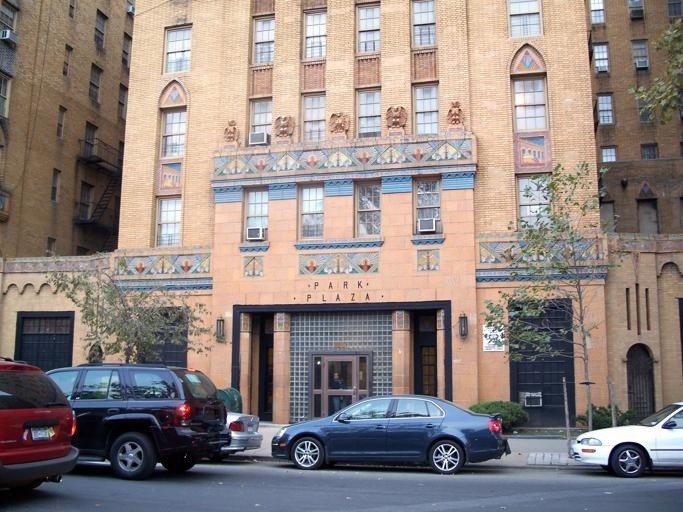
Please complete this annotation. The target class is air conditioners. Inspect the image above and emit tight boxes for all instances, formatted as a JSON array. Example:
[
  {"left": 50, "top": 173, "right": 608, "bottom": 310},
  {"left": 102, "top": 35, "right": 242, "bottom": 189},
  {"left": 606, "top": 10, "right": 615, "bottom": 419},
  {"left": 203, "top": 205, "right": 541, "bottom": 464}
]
[
  {"left": 246, "top": 228, "right": 265, "bottom": 240},
  {"left": 4, "top": 29, "right": 19, "bottom": 45},
  {"left": 525, "top": 393, "right": 542, "bottom": 408},
  {"left": 127, "top": 5, "right": 135, "bottom": 14},
  {"left": 637, "top": 60, "right": 649, "bottom": 69},
  {"left": 418, "top": 218, "right": 435, "bottom": 232},
  {"left": 598, "top": 65, "right": 606, "bottom": 71},
  {"left": 631, "top": 9, "right": 643, "bottom": 19}
]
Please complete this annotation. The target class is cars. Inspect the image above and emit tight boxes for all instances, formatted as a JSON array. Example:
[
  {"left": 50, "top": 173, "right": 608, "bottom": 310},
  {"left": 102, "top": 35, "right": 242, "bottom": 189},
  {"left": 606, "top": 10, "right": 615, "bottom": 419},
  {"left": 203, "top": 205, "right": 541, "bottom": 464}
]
[
  {"left": 272, "top": 394, "right": 511, "bottom": 474},
  {"left": 0, "top": 360, "right": 264, "bottom": 490},
  {"left": 571, "top": 402, "right": 683, "bottom": 478}
]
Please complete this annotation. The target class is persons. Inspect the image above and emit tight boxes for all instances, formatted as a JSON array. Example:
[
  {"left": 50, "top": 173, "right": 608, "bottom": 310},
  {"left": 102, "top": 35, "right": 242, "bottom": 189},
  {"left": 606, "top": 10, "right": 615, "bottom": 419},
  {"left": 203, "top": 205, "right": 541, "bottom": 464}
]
[{"left": 329, "top": 372, "right": 347, "bottom": 414}]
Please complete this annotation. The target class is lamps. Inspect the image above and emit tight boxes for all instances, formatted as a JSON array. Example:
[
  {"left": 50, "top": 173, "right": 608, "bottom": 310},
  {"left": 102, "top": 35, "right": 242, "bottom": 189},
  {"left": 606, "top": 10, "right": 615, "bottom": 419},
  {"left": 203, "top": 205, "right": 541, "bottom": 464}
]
[
  {"left": 459, "top": 313, "right": 468, "bottom": 336},
  {"left": 215, "top": 317, "right": 224, "bottom": 340}
]
